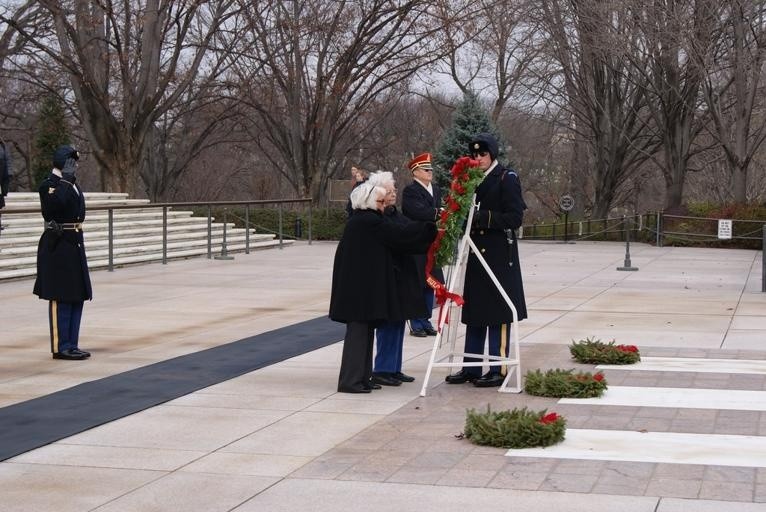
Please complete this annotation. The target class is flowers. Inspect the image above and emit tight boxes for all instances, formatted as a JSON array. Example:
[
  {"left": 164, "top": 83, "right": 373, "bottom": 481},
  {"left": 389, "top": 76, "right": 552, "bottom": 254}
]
[{"left": 433, "top": 158, "right": 482, "bottom": 270}]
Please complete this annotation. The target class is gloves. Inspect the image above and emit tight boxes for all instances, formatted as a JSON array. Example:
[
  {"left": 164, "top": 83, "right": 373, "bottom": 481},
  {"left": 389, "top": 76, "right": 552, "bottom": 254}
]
[
  {"left": 59, "top": 156, "right": 79, "bottom": 177},
  {"left": 473, "top": 209, "right": 490, "bottom": 228}
]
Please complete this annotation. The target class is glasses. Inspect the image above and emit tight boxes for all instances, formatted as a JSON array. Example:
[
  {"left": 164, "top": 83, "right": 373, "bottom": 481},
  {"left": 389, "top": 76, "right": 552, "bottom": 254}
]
[
  {"left": 376, "top": 197, "right": 385, "bottom": 205},
  {"left": 473, "top": 152, "right": 490, "bottom": 158},
  {"left": 422, "top": 169, "right": 433, "bottom": 173},
  {"left": 385, "top": 187, "right": 398, "bottom": 196}
]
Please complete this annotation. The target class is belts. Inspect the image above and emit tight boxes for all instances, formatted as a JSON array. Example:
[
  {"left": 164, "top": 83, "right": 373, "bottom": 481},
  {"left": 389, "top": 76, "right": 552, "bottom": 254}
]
[{"left": 46, "top": 221, "right": 84, "bottom": 233}]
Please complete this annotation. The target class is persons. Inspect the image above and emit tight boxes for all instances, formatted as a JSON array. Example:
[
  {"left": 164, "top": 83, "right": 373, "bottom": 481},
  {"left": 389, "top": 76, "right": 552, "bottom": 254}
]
[
  {"left": 369, "top": 170, "right": 443, "bottom": 386},
  {"left": 33, "top": 145, "right": 92, "bottom": 360},
  {"left": 401, "top": 153, "right": 445, "bottom": 337},
  {"left": 328, "top": 183, "right": 445, "bottom": 394},
  {"left": 446, "top": 134, "right": 529, "bottom": 388},
  {"left": 345, "top": 169, "right": 369, "bottom": 218},
  {"left": 0, "top": 137, "right": 14, "bottom": 230},
  {"left": 351, "top": 165, "right": 361, "bottom": 187}
]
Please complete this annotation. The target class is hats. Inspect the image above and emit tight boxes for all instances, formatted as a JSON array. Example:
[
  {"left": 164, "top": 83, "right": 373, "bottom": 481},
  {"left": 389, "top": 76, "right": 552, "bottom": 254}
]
[
  {"left": 467, "top": 132, "right": 499, "bottom": 163},
  {"left": 51, "top": 144, "right": 81, "bottom": 164},
  {"left": 406, "top": 152, "right": 435, "bottom": 173}
]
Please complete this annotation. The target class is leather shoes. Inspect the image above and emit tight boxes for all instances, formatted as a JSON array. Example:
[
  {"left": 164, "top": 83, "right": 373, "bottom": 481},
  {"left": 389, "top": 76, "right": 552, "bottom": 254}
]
[
  {"left": 51, "top": 347, "right": 86, "bottom": 361},
  {"left": 370, "top": 371, "right": 404, "bottom": 387},
  {"left": 409, "top": 327, "right": 428, "bottom": 338},
  {"left": 422, "top": 325, "right": 438, "bottom": 337},
  {"left": 70, "top": 346, "right": 91, "bottom": 358},
  {"left": 390, "top": 369, "right": 415, "bottom": 383},
  {"left": 444, "top": 367, "right": 483, "bottom": 385},
  {"left": 472, "top": 369, "right": 508, "bottom": 388},
  {"left": 362, "top": 379, "right": 382, "bottom": 390},
  {"left": 337, "top": 382, "right": 372, "bottom": 394}
]
